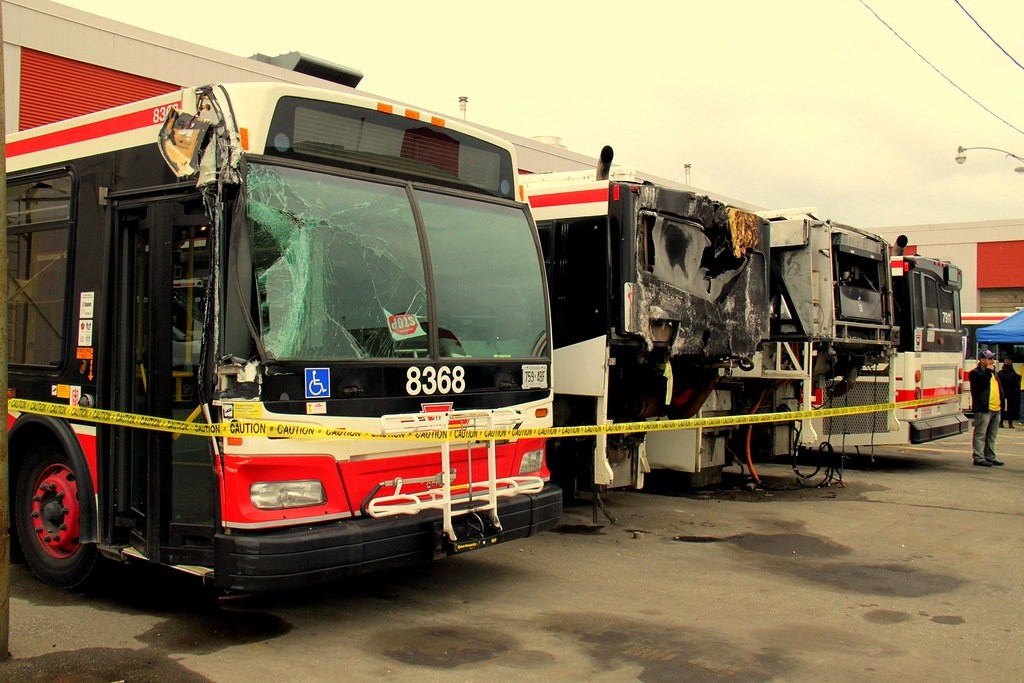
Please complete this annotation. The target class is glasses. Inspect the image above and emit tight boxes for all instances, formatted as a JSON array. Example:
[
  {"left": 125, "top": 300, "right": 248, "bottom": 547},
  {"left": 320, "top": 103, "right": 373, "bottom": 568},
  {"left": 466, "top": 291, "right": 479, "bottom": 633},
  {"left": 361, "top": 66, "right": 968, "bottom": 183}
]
[{"left": 984, "top": 357, "right": 996, "bottom": 360}]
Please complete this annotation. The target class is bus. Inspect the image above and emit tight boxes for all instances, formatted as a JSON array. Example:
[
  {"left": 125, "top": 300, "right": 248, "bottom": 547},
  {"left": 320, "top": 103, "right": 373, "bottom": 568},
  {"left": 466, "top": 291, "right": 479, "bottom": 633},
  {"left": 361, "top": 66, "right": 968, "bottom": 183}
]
[
  {"left": 960, "top": 312, "right": 1024, "bottom": 414},
  {"left": 769, "top": 206, "right": 900, "bottom": 457},
  {"left": 6, "top": 81, "right": 553, "bottom": 603},
  {"left": 517, "top": 143, "right": 769, "bottom": 487},
  {"left": 887, "top": 254, "right": 969, "bottom": 444}
]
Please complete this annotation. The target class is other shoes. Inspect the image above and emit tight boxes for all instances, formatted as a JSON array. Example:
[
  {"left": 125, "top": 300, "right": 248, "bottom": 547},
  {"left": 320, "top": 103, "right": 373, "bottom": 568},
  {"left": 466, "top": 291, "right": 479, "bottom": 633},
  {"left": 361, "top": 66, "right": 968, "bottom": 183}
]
[
  {"left": 986, "top": 458, "right": 1004, "bottom": 465},
  {"left": 1009, "top": 425, "right": 1014, "bottom": 428},
  {"left": 974, "top": 460, "right": 993, "bottom": 466},
  {"left": 999, "top": 424, "right": 1004, "bottom": 428}
]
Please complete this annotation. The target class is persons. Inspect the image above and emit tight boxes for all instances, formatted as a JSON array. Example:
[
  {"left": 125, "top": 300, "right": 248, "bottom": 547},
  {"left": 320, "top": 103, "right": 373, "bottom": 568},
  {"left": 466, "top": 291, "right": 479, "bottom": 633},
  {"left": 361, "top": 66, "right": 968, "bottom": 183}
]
[
  {"left": 997, "top": 359, "right": 1018, "bottom": 428},
  {"left": 968, "top": 350, "right": 1005, "bottom": 466},
  {"left": 1017, "top": 366, "right": 1024, "bottom": 425}
]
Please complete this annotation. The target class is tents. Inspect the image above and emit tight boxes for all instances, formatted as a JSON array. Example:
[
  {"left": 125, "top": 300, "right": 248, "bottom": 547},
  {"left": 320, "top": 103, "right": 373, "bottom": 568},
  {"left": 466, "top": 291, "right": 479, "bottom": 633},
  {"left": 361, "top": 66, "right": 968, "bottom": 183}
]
[{"left": 976, "top": 310, "right": 1024, "bottom": 367}]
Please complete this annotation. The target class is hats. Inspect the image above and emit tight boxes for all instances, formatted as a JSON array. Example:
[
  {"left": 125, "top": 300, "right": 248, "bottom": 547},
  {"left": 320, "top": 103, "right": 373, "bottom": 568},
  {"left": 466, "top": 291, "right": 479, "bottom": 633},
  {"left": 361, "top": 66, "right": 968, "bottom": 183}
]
[{"left": 978, "top": 349, "right": 997, "bottom": 360}]
